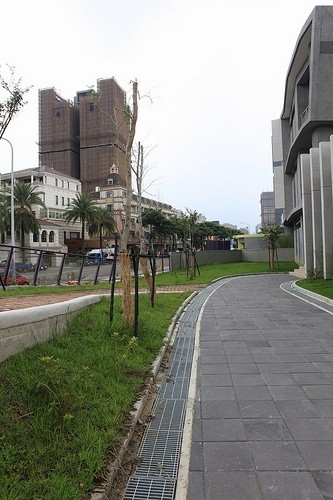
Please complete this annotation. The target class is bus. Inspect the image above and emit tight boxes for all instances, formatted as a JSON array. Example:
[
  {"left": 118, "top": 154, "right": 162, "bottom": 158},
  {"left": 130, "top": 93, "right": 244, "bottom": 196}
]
[{"left": 230, "top": 234, "right": 272, "bottom": 250}]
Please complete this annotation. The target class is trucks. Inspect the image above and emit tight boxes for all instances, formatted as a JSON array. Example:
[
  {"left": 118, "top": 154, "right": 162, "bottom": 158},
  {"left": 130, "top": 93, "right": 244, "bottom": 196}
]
[
  {"left": 0, "top": 258, "right": 33, "bottom": 273},
  {"left": 86, "top": 252, "right": 114, "bottom": 265}
]
